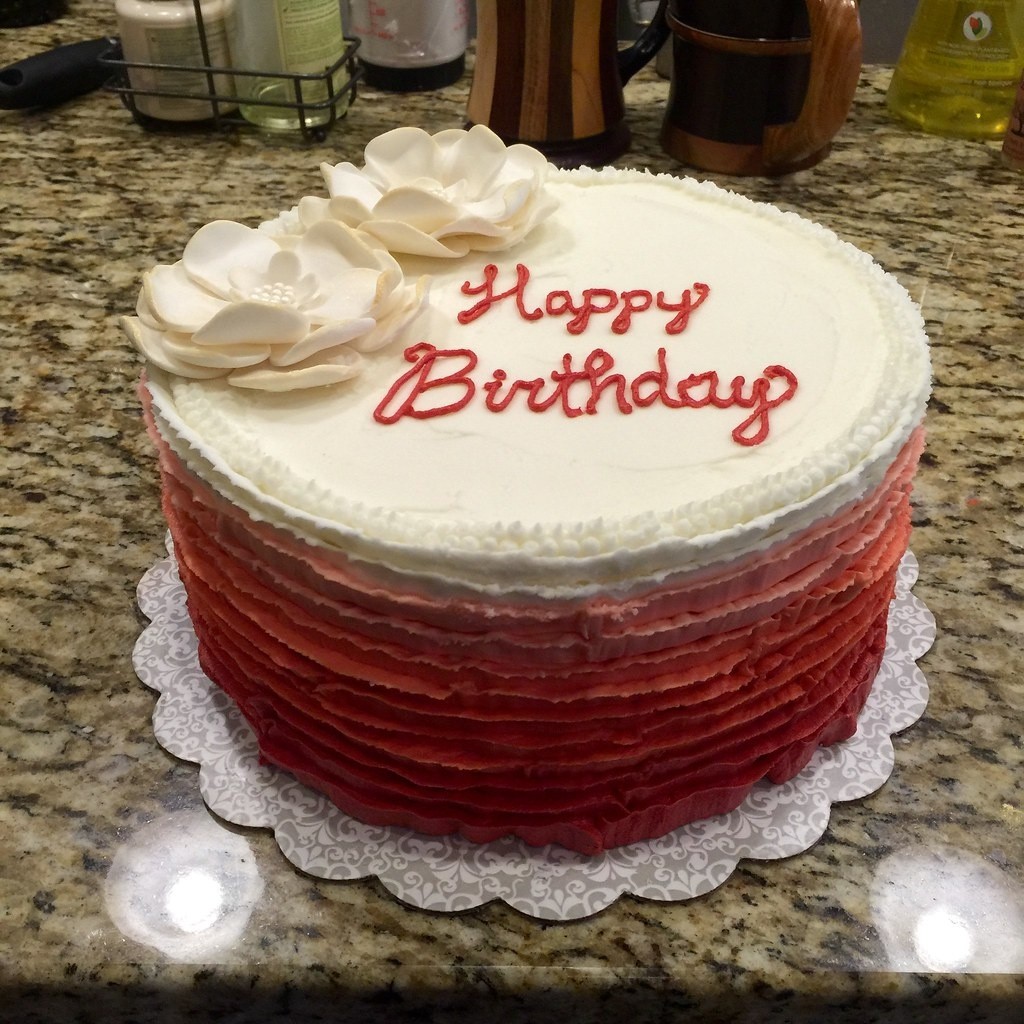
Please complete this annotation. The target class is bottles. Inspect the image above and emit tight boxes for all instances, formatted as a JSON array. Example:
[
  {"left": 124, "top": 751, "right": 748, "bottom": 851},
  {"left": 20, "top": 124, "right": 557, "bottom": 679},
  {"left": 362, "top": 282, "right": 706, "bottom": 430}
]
[{"left": 882, "top": 0, "right": 1024, "bottom": 145}]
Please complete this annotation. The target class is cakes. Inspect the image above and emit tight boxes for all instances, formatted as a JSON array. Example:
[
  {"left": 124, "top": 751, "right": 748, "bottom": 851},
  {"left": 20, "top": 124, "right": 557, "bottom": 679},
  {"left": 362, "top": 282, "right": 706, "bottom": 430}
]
[{"left": 117, "top": 125, "right": 933, "bottom": 855}]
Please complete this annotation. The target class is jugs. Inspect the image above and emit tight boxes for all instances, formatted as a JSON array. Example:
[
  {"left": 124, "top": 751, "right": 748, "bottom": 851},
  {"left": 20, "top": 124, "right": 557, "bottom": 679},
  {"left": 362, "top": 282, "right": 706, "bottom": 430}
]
[
  {"left": 659, "top": 1, "right": 863, "bottom": 177},
  {"left": 461, "top": 0, "right": 672, "bottom": 171}
]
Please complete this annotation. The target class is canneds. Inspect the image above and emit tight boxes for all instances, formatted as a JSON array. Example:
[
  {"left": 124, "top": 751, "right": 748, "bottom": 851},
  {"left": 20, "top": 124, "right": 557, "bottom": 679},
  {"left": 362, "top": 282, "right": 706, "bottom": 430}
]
[{"left": 113, "top": 0, "right": 351, "bottom": 129}]
